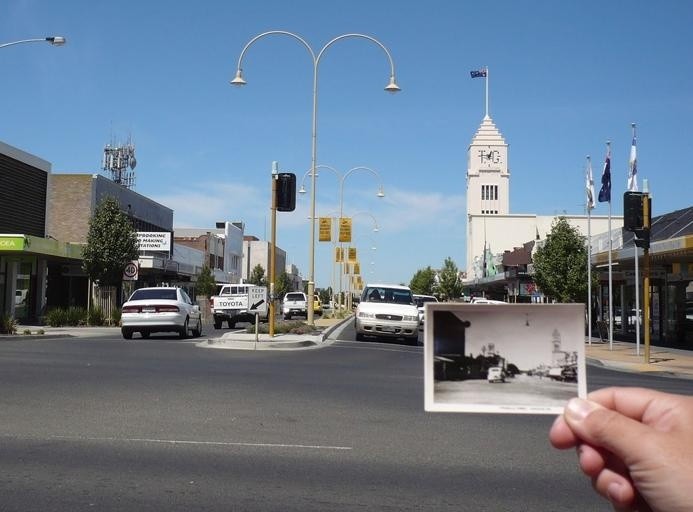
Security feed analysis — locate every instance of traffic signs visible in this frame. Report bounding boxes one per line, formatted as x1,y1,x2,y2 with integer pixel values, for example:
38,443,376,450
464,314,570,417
247,286,267,312
124,263,137,277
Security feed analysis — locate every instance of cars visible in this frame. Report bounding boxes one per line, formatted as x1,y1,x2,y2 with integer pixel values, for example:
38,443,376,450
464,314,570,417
14,287,28,305
487,367,507,384
352,284,510,345
120,287,202,340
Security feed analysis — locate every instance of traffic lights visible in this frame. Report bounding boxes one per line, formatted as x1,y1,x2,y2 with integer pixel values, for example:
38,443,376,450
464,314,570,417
635,222,650,249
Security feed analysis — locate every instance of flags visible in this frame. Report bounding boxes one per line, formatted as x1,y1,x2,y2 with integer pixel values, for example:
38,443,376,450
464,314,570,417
626,135,637,192
597,150,612,203
470,68,486,79
585,162,596,210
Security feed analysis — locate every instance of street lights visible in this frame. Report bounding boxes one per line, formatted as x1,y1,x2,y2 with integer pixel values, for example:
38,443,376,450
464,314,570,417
300,164,385,317
231,31,403,336
1,33,69,59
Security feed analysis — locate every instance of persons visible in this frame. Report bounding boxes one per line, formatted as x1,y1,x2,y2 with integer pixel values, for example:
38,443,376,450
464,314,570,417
548,384,693,512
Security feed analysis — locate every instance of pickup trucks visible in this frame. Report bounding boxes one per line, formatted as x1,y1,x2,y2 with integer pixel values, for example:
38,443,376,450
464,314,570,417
210,282,270,326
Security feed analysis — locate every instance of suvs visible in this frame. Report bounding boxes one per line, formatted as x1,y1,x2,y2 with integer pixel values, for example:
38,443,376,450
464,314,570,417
278,290,324,319
